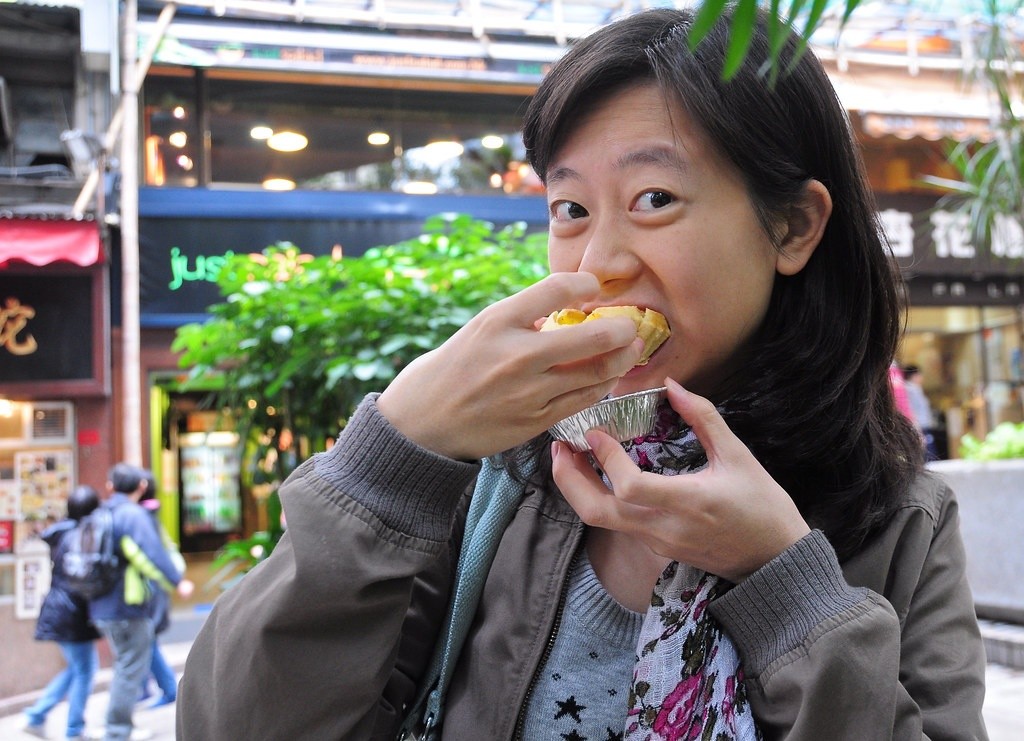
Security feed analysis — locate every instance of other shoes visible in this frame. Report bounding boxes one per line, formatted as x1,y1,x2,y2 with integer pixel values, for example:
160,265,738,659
65,735,99,741
149,694,176,709
21,723,53,741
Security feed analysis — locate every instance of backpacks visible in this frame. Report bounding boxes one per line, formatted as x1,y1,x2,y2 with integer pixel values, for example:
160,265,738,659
54,500,129,599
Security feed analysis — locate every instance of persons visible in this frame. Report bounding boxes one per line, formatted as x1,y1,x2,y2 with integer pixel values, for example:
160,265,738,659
903,366,933,460
24,464,195,741
175,9,985,741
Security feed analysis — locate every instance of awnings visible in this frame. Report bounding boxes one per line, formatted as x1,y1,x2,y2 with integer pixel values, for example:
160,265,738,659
0,218,106,269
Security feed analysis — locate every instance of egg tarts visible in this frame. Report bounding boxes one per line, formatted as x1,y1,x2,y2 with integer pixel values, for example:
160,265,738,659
535,306,672,366
